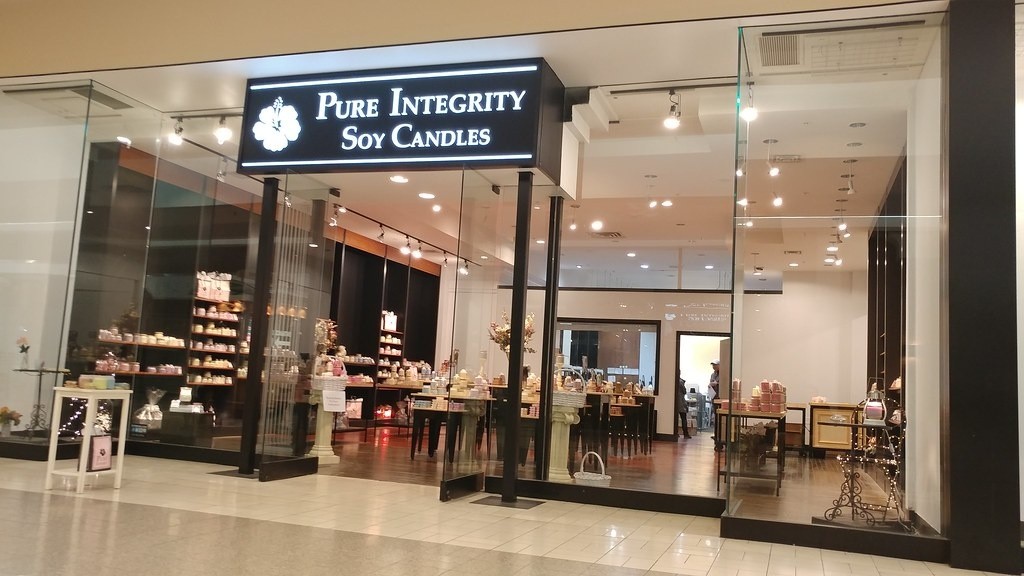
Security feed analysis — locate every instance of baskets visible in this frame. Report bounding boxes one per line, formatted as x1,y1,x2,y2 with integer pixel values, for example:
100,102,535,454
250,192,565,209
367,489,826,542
552,369,587,409
311,354,348,390
573,451,611,487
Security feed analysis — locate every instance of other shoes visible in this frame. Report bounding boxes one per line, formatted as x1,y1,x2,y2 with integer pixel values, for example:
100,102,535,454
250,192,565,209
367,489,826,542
683,435,691,439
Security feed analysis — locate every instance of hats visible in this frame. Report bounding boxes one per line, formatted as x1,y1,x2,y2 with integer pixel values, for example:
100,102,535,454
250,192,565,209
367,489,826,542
711,360,722,366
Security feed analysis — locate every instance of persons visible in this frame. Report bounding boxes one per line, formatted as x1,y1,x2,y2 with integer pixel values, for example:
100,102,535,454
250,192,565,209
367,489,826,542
708,358,721,443
676,369,692,439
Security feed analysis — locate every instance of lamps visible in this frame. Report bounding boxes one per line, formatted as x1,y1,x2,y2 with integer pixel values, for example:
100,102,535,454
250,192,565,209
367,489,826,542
168,117,185,146
377,224,385,242
834,255,842,266
442,251,448,268
738,82,759,123
400,236,412,255
459,260,470,276
338,207,347,214
835,232,843,245
848,159,856,196
844,230,851,239
836,201,848,231
412,240,423,258
663,89,683,131
211,116,232,147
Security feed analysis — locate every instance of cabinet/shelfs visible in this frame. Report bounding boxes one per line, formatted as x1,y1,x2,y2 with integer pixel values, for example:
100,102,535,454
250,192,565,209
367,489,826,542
65,148,441,438
